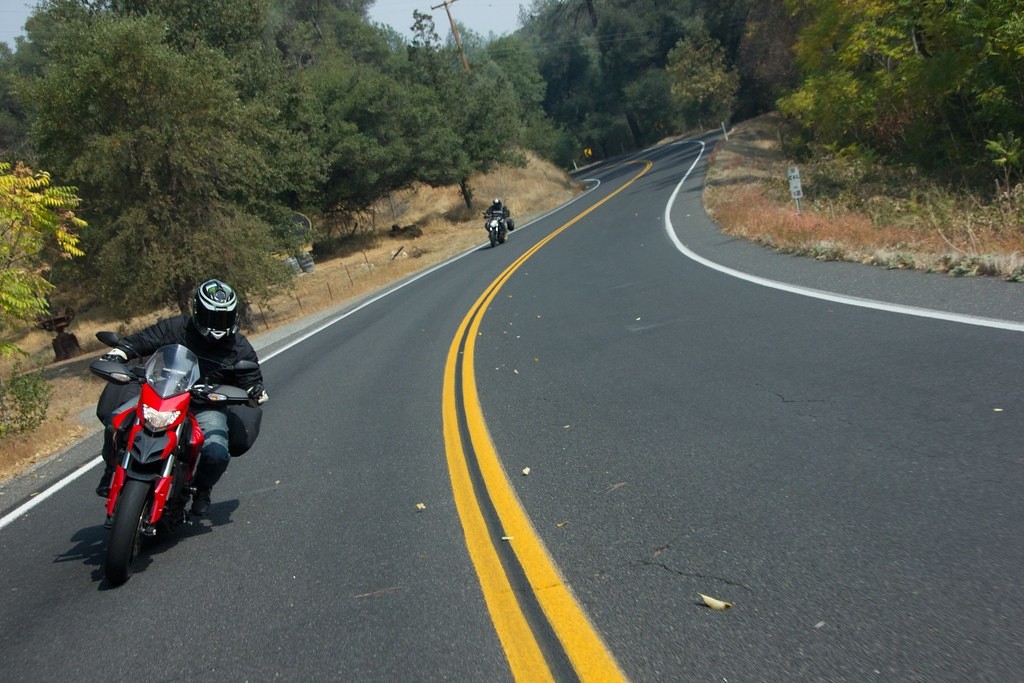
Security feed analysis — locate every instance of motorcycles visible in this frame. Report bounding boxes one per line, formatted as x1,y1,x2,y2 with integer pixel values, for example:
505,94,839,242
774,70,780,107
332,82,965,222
90,331,257,584
483,211,514,247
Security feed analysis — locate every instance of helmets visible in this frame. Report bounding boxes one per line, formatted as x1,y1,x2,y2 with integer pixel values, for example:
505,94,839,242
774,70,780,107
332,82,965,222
193,279,238,339
493,199,501,208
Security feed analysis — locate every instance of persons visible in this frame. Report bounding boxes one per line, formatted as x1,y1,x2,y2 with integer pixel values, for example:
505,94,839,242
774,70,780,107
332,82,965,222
484,198,508,240
100,279,269,515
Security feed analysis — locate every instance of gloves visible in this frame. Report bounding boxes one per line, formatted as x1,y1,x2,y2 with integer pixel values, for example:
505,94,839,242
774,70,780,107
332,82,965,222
99,347,129,364
245,384,269,403
503,215,507,218
484,216,487,219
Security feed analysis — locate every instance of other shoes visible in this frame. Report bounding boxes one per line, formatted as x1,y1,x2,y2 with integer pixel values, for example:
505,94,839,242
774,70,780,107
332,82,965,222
190,491,210,516
96,473,113,496
504,233,509,240
